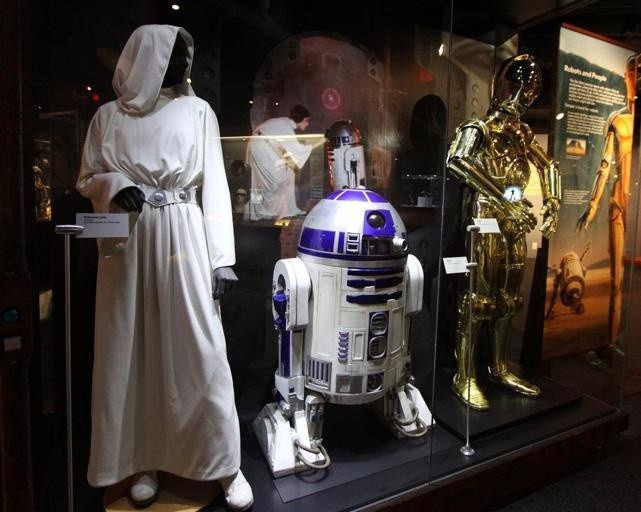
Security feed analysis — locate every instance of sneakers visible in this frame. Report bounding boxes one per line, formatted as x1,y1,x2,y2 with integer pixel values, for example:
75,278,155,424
128,471,162,507
220,469,256,509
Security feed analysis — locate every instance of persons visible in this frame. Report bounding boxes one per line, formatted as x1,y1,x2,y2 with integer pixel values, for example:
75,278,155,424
445,53,564,411
572,55,636,372
237,106,329,220
75,24,255,509
